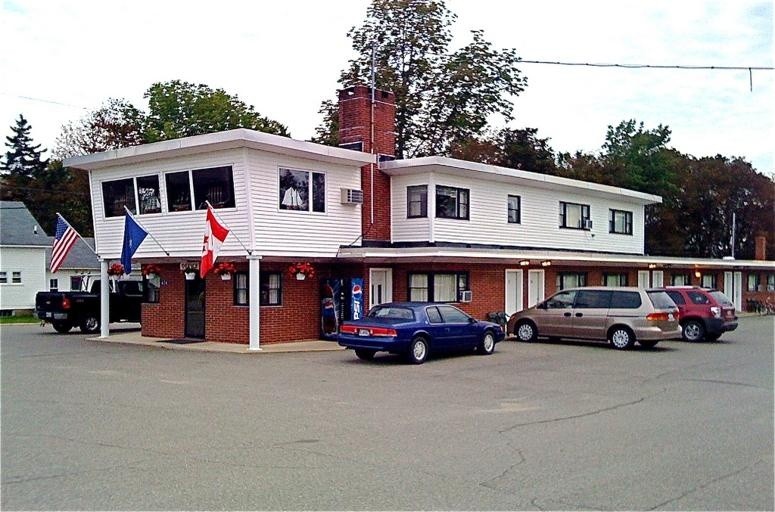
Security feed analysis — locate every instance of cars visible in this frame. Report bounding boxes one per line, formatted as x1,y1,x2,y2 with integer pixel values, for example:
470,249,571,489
665,285,739,343
338,300,505,364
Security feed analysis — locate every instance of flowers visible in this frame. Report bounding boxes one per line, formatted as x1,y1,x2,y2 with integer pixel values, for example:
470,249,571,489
213,262,237,277
107,263,124,276
141,263,160,275
286,260,316,278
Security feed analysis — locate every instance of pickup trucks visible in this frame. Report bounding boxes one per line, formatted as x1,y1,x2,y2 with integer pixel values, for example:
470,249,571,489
35,279,161,336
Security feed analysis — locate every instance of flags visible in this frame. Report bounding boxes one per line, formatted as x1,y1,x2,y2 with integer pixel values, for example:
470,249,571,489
121,212,149,275
49,217,79,274
199,208,231,278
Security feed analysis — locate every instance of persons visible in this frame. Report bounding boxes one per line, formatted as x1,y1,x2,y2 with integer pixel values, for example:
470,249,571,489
764,295,773,312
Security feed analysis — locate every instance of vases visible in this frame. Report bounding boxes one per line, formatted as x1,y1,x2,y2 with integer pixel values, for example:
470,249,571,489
221,272,231,280
111,274,121,280
146,273,155,279
295,272,306,280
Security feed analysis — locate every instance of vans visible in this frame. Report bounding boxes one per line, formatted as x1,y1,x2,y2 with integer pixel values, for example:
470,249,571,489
506,286,683,349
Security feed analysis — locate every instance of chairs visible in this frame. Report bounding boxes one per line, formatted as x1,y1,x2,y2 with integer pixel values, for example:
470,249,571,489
487,313,505,332
497,311,510,332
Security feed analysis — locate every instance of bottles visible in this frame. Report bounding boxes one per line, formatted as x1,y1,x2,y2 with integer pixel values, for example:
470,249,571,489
321,281,336,335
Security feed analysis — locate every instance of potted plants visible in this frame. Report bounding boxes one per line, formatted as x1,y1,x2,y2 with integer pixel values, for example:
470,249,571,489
182,268,199,280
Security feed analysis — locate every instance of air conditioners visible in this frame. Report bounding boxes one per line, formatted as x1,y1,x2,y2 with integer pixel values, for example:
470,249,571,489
583,220,593,229
459,291,472,303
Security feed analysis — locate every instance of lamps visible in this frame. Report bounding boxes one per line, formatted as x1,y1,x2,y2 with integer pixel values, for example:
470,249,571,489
695,272,701,281
519,260,530,266
540,260,552,268
648,263,657,269
